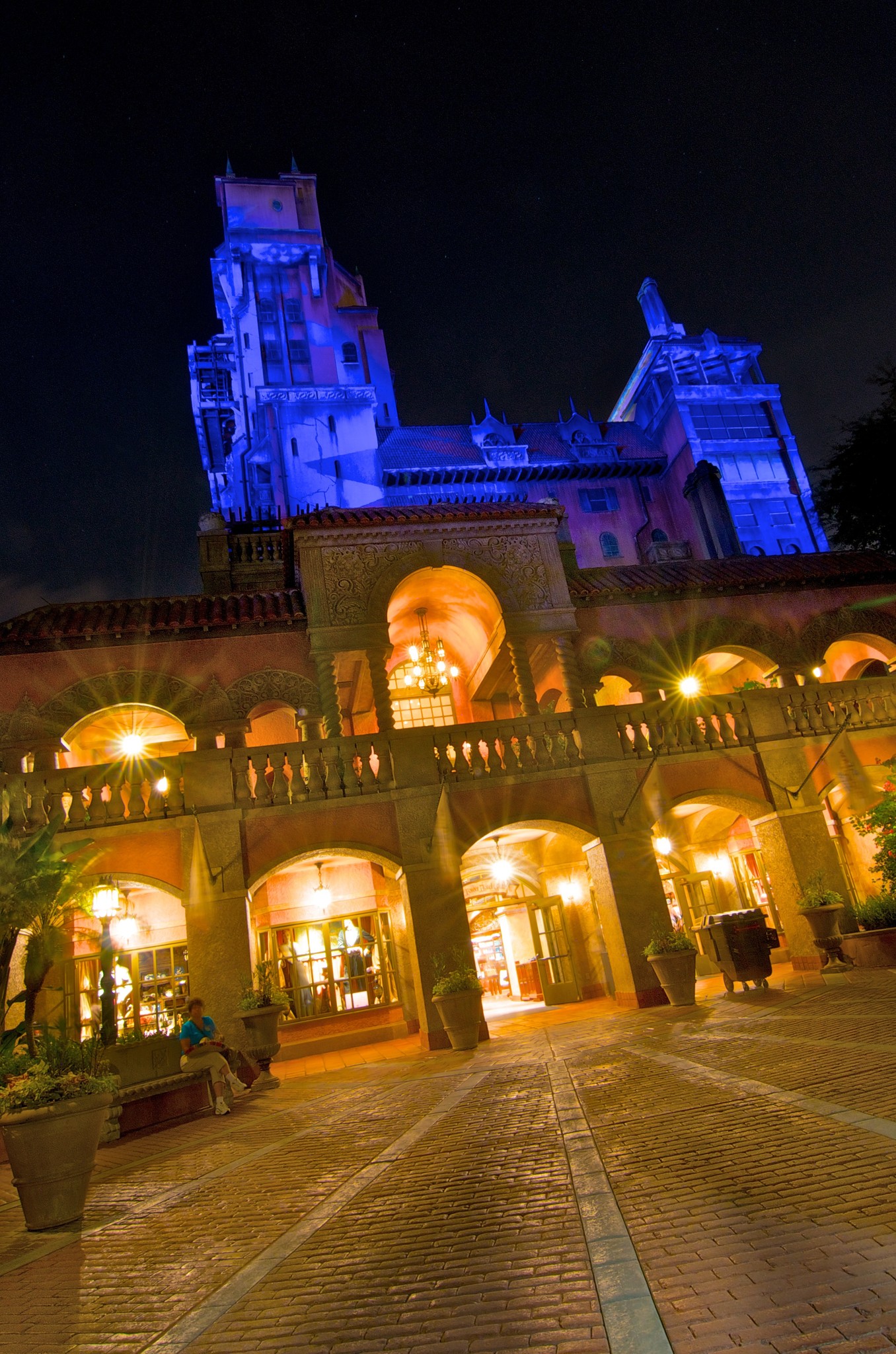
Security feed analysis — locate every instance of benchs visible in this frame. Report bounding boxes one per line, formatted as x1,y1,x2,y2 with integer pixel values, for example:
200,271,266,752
99,1060,241,1142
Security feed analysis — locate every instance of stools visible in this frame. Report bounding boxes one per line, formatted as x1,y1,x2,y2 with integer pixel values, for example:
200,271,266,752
488,975,502,996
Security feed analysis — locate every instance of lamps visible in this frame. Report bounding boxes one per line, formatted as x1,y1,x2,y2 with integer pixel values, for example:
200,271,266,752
404,607,459,699
314,862,326,891
117,889,137,946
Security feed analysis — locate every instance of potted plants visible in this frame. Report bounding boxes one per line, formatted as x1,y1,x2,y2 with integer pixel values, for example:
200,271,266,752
0,1059,120,1230
641,913,698,1006
429,946,488,1050
791,870,853,974
233,959,293,1091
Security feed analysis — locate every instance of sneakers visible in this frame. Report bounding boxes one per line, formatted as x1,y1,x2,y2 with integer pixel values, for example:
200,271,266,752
215,1102,230,1115
231,1078,248,1091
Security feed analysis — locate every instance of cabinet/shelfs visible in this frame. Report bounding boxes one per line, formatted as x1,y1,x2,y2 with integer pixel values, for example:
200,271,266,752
515,960,552,1002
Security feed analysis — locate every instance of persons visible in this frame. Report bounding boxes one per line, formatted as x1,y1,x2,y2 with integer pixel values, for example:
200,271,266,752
180,996,248,1115
98,956,132,1037
280,929,315,1018
337,918,375,994
